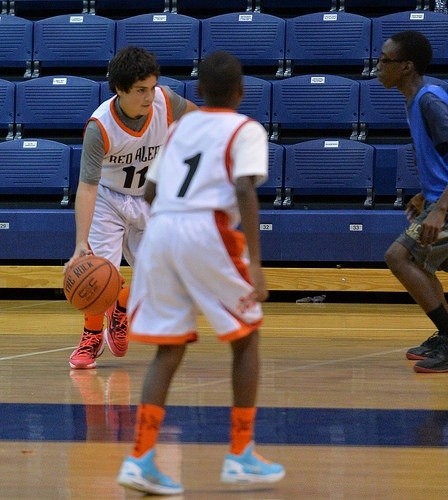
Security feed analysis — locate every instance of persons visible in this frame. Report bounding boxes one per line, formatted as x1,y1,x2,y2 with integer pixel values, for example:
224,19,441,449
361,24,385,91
60,45,199,371
114,47,290,495
373,30,448,373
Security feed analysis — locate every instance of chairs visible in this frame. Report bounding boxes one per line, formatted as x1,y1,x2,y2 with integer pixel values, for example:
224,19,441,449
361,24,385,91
0,0,448,209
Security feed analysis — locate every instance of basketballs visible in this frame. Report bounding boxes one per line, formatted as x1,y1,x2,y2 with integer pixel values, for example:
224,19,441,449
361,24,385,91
63,256,120,314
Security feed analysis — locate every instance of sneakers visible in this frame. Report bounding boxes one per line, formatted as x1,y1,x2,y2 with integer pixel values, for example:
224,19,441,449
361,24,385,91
69,324,105,369
103,287,131,357
70,369,110,406
414,342,448,373
119,449,184,494
220,442,286,484
105,371,134,426
406,330,441,359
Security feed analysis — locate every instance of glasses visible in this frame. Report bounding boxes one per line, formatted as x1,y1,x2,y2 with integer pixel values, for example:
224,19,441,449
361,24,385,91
377,52,404,68
